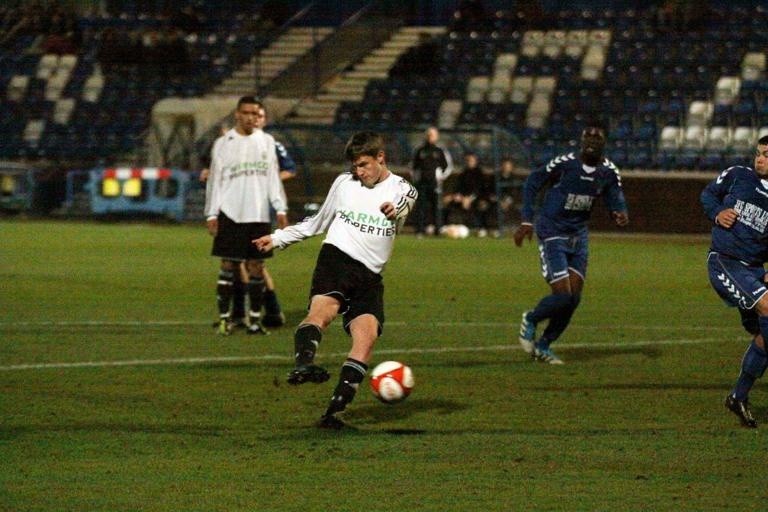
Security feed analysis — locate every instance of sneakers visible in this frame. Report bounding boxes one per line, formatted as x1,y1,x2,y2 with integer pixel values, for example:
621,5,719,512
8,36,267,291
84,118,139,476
518,311,535,356
225,313,250,328
725,392,759,428
285,363,331,385
260,312,285,327
248,310,261,335
211,313,233,336
534,347,564,366
316,414,346,430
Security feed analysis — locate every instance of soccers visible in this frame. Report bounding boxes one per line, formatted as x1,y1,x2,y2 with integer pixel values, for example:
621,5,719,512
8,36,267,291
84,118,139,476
370,361,415,405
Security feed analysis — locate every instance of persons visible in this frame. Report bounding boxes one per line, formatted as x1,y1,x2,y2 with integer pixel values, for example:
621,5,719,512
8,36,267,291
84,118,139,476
440,151,523,239
516,125,630,366
410,129,453,234
701,136,768,429
251,132,418,411
199,102,296,326
255,104,298,182
204,97,289,336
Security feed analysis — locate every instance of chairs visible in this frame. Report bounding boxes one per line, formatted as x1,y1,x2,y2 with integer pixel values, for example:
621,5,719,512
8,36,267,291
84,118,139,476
497,54,516,68
589,31,600,43
759,128,768,139
565,46,580,56
55,99,75,109
87,76,102,88
588,45,602,55
743,53,765,80
698,155,723,172
665,154,679,171
467,92,481,102
510,91,526,103
53,113,68,124
441,101,461,115
567,31,578,44
84,89,98,102
600,31,611,44
57,70,68,79
732,127,757,151
489,91,504,102
723,155,747,170
512,78,532,90
544,33,554,43
469,78,488,90
532,31,543,43
581,69,598,80
577,31,586,44
524,31,532,43
7,90,20,101
533,93,548,99
535,77,555,89
526,116,543,128
716,77,741,105
37,69,50,78
661,126,683,148
39,134,63,158
684,126,707,149
494,69,510,79
583,55,603,66
492,79,510,90
11,77,27,87
530,100,549,111
439,116,454,128
709,127,731,150
46,90,60,101
522,46,538,56
554,31,565,43
59,56,76,69
679,154,698,172
48,78,64,88
543,46,558,56
23,121,43,140
41,56,58,67
688,101,714,126
